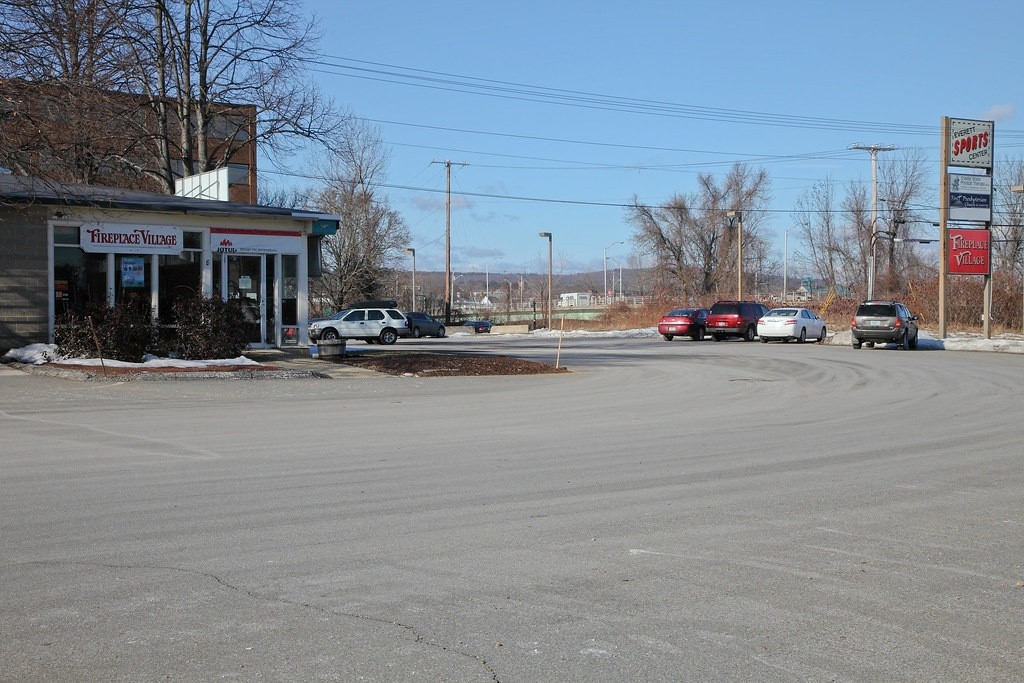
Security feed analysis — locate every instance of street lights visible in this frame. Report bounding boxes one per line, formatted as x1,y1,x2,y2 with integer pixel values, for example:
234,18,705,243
727,211,743,302
404,247,416,312
783,221,808,303
537,231,551,332
606,257,622,301
604,240,625,306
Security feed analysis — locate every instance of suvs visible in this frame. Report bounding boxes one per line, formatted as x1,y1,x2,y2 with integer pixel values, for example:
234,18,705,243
850,299,920,351
307,300,410,346
706,301,770,342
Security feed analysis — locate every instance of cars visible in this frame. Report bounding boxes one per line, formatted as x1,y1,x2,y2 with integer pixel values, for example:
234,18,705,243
474,322,491,333
404,312,446,338
757,308,826,343
657,308,712,341
